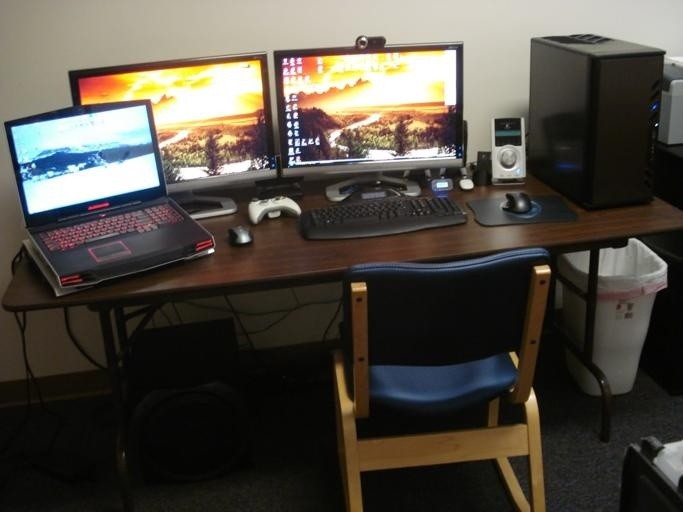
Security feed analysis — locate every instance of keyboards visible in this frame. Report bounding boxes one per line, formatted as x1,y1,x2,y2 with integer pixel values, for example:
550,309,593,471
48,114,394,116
299,195,467,241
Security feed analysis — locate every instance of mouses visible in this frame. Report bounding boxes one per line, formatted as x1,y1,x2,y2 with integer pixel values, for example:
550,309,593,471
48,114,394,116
228,224,254,246
506,190,533,214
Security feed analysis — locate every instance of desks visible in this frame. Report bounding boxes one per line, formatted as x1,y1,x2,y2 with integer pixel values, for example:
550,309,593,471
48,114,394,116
1,170,683,512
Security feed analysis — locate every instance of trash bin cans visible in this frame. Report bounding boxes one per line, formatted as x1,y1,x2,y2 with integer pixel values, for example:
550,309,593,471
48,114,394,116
559,238,668,397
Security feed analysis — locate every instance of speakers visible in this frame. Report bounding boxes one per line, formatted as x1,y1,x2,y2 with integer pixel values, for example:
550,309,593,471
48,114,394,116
529,34,666,212
491,116,527,186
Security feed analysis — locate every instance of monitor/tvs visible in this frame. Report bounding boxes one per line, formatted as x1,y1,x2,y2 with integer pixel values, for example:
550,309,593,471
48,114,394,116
67,51,278,221
274,42,465,202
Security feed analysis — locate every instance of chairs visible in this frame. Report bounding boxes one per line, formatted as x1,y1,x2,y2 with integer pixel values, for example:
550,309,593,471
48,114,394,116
330,246,558,512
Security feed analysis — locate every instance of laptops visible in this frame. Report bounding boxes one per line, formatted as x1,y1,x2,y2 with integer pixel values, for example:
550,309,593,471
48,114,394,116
4,99,217,289
